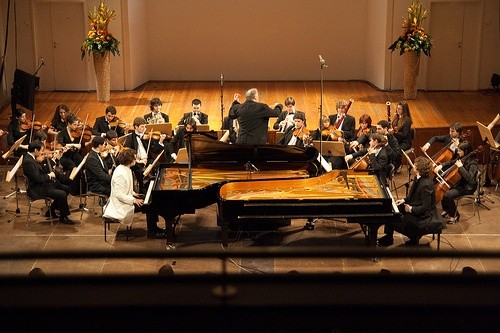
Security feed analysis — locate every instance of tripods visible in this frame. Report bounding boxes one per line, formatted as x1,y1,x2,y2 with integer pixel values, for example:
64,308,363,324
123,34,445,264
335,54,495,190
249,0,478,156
460,121,500,210
3,133,97,222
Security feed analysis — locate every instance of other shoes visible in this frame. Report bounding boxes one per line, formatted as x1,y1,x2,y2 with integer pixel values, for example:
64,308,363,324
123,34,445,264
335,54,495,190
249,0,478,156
379,235,393,245
405,238,419,247
147,227,167,239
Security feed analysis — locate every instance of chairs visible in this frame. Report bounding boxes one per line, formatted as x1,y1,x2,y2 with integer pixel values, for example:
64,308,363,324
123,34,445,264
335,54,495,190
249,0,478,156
22,173,54,228
79,169,105,222
400,128,417,172
455,169,481,221
98,195,131,242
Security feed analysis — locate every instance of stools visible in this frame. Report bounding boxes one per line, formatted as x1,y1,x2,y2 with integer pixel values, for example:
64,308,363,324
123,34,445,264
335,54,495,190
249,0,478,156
422,209,445,251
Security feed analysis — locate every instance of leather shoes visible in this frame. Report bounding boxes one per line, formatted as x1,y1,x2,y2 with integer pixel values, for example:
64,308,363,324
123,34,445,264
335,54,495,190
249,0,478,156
59,217,75,225
44,212,60,218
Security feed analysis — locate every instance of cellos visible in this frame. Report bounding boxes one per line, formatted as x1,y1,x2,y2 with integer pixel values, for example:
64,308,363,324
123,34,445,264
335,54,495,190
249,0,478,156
488,146,500,192
434,145,484,205
432,129,472,179
349,139,388,170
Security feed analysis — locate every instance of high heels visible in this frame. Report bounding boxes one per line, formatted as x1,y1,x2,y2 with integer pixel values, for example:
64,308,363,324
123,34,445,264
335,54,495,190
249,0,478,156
441,212,448,217
447,214,460,224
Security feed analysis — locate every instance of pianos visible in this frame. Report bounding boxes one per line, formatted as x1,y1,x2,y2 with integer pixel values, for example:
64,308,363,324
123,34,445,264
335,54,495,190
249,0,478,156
141,144,319,245
217,169,403,248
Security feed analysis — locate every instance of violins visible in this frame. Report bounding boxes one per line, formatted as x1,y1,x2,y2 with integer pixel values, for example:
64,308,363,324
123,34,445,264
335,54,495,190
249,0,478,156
70,127,92,140
22,119,55,129
142,130,174,140
36,142,130,163
108,117,133,128
322,125,349,145
356,128,371,138
293,127,313,143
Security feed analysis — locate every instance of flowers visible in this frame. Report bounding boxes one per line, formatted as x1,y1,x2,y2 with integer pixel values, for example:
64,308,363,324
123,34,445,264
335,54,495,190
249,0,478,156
78,0,123,65
387,0,435,61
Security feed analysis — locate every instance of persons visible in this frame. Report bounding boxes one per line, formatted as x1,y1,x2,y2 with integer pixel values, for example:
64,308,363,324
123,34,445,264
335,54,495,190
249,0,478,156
220,87,283,145
7,104,93,196
309,99,401,183
388,101,411,174
85,105,197,196
102,148,167,238
175,98,208,129
23,141,74,224
422,122,479,223
278,113,312,147
377,157,436,245
273,96,306,132
144,97,169,124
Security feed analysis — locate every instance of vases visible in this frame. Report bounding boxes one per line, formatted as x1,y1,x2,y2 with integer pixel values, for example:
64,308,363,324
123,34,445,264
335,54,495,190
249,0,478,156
403,50,418,99
91,50,112,104
